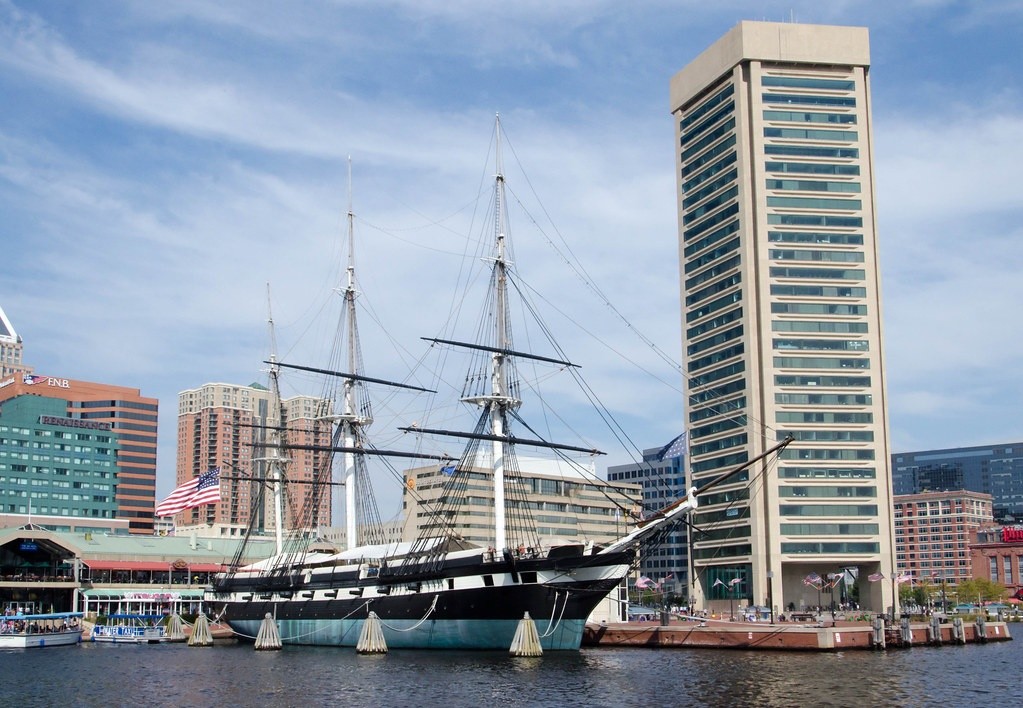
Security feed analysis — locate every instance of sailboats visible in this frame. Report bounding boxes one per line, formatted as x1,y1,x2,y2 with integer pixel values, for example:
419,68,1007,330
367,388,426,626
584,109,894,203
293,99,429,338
199,112,798,660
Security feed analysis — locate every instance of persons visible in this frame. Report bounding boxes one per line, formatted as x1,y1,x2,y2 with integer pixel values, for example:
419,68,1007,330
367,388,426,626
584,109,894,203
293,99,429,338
0,616,79,635
644,600,943,630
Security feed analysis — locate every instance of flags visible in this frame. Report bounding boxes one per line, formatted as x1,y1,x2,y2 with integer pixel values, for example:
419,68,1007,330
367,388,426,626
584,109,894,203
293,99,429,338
632,567,944,594
156,466,222,517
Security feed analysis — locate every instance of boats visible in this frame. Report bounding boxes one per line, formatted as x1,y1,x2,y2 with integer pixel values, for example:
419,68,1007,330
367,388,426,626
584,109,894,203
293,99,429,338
0,611,84,649
89,614,172,645
181,623,236,639
